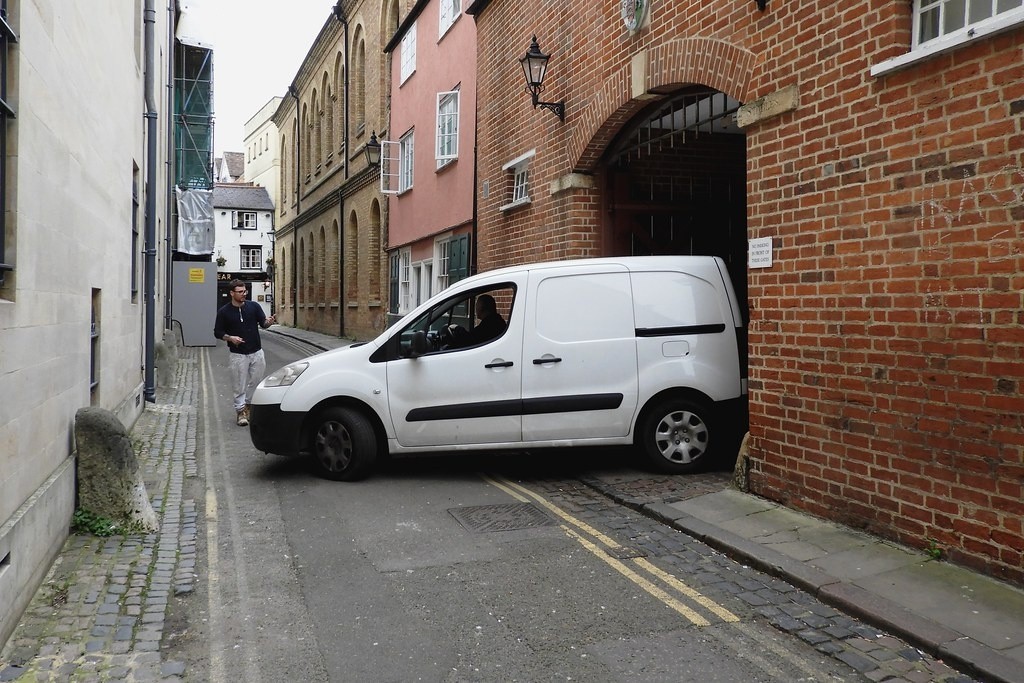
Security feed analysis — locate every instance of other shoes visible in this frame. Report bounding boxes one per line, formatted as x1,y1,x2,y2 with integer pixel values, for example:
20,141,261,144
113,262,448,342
245,404,250,420
237,411,249,426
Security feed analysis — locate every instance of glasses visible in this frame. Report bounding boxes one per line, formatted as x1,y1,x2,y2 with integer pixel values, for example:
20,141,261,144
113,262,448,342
234,290,249,295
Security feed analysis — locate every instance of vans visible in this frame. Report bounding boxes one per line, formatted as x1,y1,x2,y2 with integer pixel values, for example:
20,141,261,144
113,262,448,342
248,256,746,481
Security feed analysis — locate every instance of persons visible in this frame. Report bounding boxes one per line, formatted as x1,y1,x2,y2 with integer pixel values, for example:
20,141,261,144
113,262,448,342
214,279,276,426
448,294,507,351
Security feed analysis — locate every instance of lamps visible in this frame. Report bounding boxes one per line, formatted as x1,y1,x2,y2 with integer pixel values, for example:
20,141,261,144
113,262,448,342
363,132,382,166
518,35,566,122
